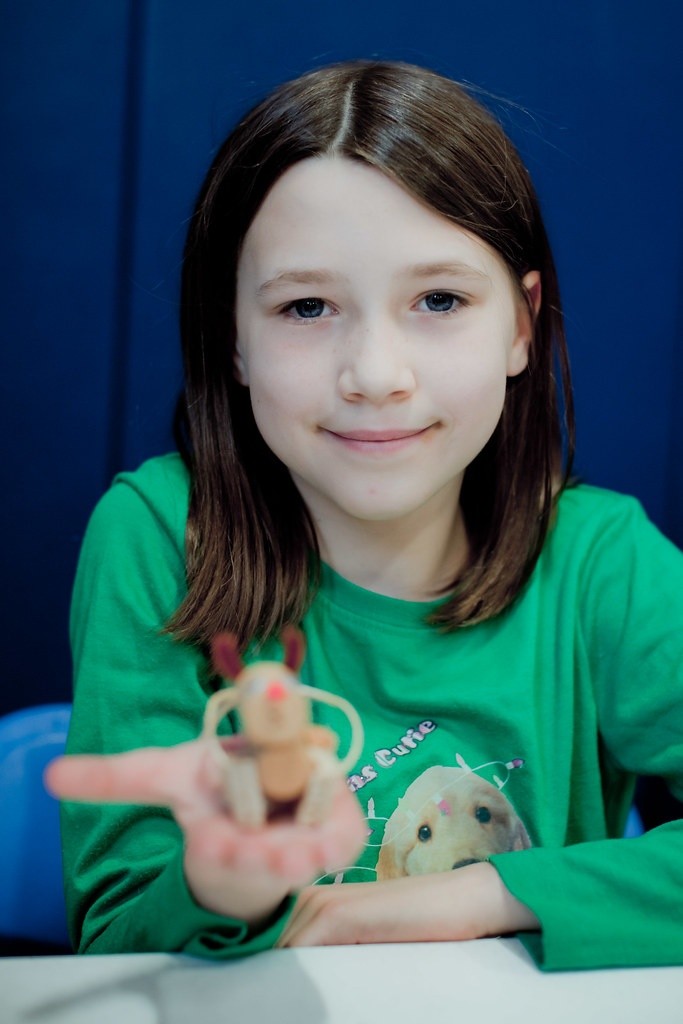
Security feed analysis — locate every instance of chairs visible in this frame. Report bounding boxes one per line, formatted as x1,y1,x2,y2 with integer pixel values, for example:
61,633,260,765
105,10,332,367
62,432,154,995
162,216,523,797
0,704,78,957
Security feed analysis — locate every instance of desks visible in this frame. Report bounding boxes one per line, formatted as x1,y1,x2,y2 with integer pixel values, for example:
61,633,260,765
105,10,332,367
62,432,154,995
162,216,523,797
0,938,683,1024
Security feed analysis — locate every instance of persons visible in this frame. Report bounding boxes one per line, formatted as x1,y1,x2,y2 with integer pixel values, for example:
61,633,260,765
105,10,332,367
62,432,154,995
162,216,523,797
46,60,683,971
